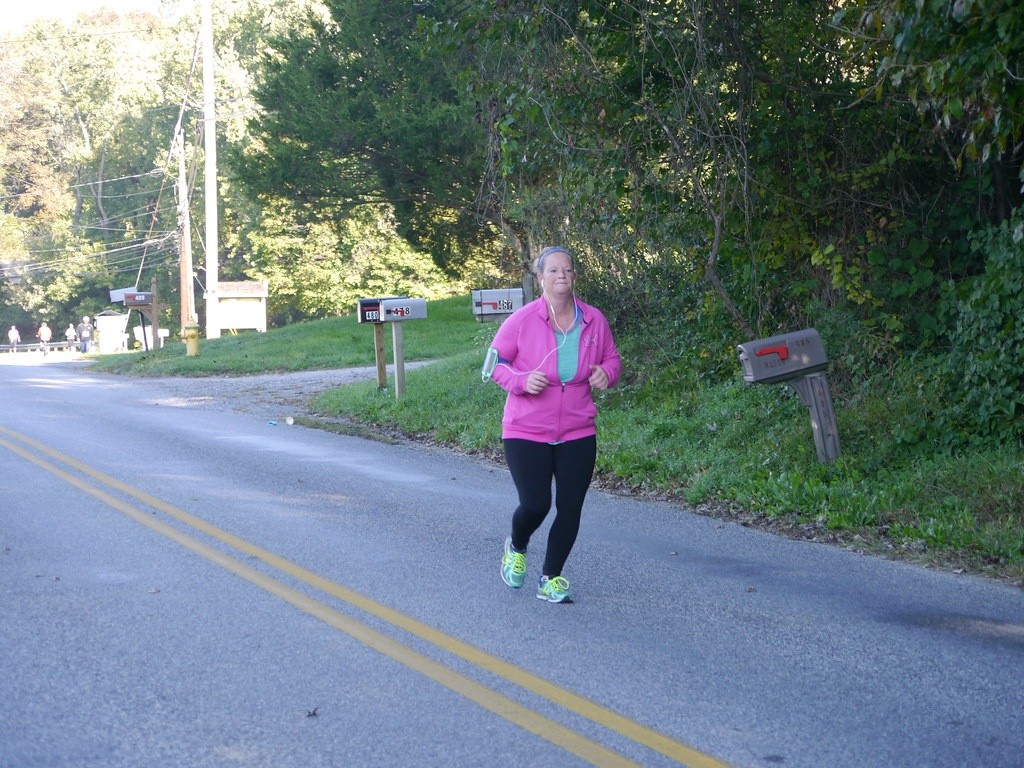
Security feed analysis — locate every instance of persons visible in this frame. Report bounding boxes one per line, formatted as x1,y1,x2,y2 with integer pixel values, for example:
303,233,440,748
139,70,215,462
65,323,76,351
38,322,52,354
481,247,620,603
8,325,21,354
76,316,94,354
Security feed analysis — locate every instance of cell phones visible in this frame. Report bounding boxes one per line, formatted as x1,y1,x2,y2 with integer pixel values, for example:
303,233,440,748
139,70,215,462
482,347,497,378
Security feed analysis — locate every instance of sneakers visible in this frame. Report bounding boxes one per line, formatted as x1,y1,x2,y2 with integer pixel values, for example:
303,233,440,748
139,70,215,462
537,575,572,603
500,537,527,589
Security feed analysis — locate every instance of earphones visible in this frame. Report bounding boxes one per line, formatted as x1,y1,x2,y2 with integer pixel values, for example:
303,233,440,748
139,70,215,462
541,279,545,288
573,280,575,285
